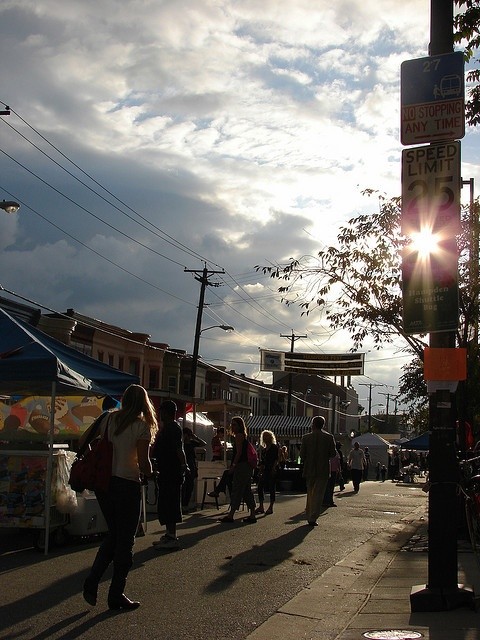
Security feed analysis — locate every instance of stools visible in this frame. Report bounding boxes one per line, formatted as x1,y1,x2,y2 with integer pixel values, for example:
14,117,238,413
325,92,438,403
200,476,221,510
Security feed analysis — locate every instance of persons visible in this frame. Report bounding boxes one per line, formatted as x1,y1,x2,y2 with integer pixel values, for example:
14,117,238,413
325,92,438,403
210,424,226,462
299,415,336,526
150,399,190,552
335,441,345,491
77,386,156,613
218,416,258,523
379,465,388,481
389,446,429,483
254,430,280,515
323,445,340,509
281,445,289,465
207,436,237,500
181,427,207,512
347,442,367,493
363,447,372,481
101,395,119,416
375,462,381,480
1,414,23,451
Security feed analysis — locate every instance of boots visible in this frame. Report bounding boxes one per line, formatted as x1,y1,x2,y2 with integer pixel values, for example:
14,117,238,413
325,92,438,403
107,575,141,611
83,552,108,606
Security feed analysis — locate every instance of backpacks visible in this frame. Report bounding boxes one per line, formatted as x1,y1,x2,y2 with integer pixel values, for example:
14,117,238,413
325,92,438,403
159,446,190,483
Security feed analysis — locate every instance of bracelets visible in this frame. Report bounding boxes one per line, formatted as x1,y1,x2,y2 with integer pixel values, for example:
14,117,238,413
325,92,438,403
181,463,189,467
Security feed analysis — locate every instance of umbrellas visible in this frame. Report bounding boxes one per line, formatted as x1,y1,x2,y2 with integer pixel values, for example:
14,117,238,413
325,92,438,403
400,433,431,450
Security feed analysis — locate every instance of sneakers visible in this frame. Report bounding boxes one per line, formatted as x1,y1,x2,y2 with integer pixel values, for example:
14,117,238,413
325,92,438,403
255,507,264,514
266,507,273,514
243,516,256,523
207,491,219,497
328,503,337,507
308,522,318,526
218,516,234,522
152,534,182,551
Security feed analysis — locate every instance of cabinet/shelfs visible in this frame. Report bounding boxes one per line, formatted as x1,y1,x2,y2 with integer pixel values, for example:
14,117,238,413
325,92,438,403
194,397,254,504
144,387,201,515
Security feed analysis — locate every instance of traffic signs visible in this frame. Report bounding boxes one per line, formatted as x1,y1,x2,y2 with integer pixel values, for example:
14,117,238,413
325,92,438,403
401,139,462,234
401,51,465,146
401,236,460,334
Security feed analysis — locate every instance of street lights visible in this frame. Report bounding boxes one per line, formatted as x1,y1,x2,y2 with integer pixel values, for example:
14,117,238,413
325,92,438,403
190,325,234,397
0,199,20,215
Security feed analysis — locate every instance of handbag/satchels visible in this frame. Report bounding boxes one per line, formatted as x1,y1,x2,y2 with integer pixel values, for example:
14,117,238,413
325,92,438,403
68,439,113,492
246,441,257,469
